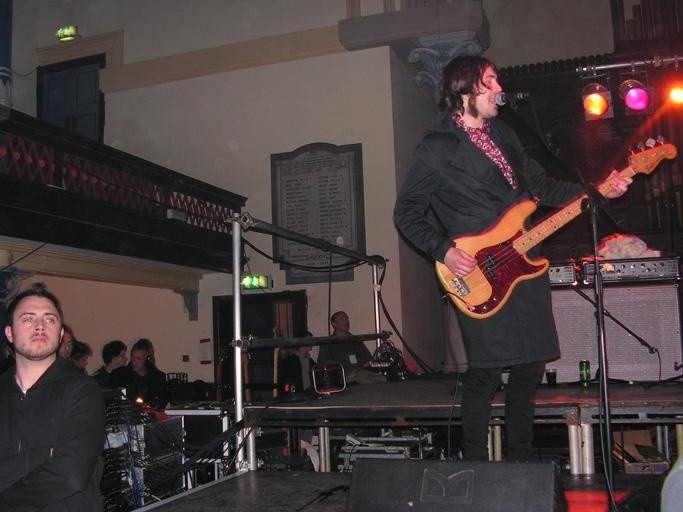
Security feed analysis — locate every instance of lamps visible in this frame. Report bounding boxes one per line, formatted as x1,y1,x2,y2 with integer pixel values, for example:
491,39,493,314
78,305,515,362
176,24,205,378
579,54,683,125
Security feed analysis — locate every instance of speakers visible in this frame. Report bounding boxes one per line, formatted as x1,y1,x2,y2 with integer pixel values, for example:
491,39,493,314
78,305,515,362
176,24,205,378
345,457,569,512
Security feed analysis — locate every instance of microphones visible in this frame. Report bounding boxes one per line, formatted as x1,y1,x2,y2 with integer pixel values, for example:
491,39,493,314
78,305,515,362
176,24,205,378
495,92,530,107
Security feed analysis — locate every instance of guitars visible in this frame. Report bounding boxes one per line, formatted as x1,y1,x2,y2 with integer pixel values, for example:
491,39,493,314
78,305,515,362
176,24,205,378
435,135,677,319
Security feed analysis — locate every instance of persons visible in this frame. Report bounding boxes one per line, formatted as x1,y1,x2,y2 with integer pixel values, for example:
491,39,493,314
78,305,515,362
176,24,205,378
0,293,103,512
55,326,74,360
318,311,372,371
279,332,316,444
71,342,91,370
393,56,634,461
92,340,130,386
111,338,170,412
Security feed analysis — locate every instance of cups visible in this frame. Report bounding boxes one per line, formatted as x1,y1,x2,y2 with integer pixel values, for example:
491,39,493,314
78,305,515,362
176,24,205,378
545,368,557,389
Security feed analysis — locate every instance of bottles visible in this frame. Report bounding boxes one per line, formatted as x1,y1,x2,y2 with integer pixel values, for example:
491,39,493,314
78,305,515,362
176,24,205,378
578,359,591,387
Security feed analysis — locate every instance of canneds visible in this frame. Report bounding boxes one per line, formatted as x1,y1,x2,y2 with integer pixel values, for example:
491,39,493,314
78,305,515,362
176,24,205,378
579,359,590,384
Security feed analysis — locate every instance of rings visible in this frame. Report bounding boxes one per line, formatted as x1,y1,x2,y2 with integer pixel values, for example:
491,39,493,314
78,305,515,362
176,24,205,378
456,273,460,278
619,189,622,195
